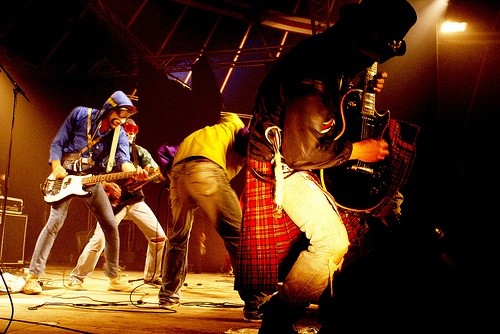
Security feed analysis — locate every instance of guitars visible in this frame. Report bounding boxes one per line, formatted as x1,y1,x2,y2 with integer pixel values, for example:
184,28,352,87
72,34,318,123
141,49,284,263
106,168,162,208
39,165,155,204
319,62,394,212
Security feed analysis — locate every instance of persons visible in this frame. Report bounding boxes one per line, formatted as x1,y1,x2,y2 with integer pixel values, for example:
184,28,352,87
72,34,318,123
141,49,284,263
69,120,167,290
159,111,266,323
23,91,150,293
239,0,419,334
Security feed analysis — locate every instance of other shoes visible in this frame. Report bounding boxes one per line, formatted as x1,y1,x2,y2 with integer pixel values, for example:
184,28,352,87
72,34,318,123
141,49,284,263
108,277,139,292
159,299,179,307
68,283,83,290
243,310,263,321
144,277,163,287
22,278,42,294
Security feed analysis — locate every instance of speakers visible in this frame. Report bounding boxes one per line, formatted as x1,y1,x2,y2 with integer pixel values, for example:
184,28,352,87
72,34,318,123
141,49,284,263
0,213,28,264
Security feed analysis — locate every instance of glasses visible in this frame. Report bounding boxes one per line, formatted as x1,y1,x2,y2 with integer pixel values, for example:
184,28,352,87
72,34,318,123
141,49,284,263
120,124,140,135
116,108,132,118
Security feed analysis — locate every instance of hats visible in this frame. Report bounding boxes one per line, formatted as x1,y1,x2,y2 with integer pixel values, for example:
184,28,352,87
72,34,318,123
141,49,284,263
338,0,417,64
